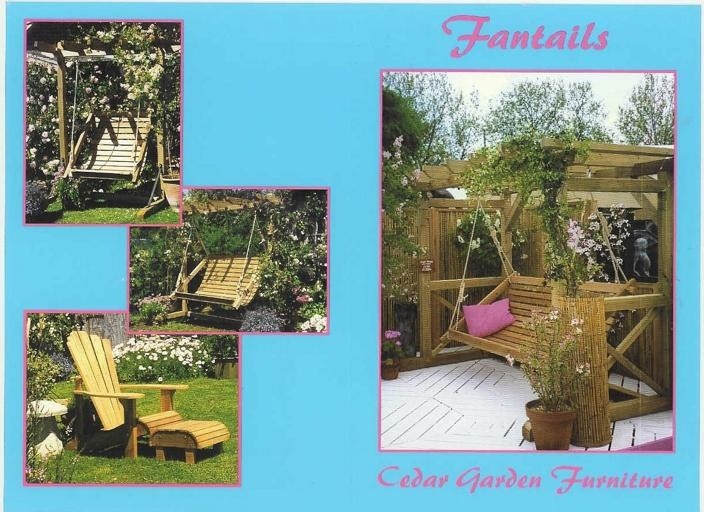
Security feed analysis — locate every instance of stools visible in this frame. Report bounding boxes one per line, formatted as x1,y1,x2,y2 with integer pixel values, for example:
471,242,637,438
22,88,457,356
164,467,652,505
147,419,231,464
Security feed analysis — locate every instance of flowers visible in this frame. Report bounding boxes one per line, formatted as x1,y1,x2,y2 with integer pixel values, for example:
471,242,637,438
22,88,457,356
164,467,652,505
453,208,529,274
566,200,631,282
381,329,405,360
504,308,594,409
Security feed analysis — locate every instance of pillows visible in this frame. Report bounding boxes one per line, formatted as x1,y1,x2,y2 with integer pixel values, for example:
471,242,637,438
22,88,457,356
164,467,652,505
461,297,516,337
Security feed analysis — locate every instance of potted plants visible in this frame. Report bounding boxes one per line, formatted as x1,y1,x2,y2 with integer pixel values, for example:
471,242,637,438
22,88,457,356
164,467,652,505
153,49,179,214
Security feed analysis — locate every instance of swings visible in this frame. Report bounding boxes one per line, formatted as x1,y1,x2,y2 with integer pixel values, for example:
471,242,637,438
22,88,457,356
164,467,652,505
432,198,635,366
62,61,153,184
171,208,268,310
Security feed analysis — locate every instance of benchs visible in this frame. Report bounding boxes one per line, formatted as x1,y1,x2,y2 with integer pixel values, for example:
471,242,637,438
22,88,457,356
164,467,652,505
433,270,636,372
59,107,150,184
165,256,261,328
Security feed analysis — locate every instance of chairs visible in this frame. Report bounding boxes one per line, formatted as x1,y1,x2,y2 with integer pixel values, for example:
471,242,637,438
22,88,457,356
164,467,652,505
64,328,188,464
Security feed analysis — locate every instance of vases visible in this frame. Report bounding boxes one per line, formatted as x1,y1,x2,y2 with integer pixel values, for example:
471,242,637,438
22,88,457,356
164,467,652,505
381,360,405,380
524,398,578,450
